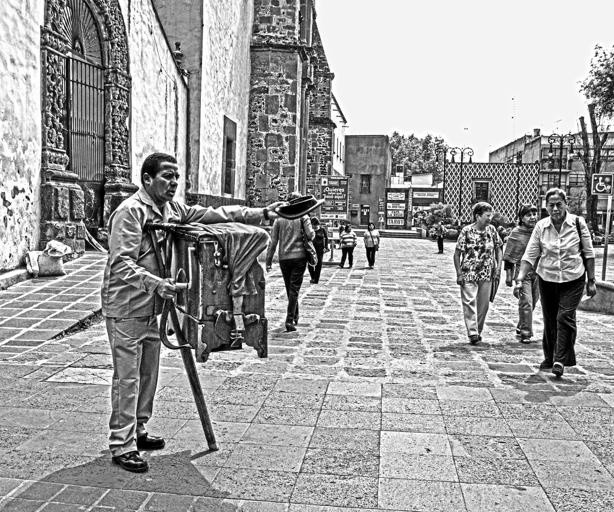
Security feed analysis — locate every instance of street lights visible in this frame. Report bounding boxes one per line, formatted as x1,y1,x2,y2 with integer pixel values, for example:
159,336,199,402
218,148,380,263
435,146,452,204
548,133,575,189
449,147,474,217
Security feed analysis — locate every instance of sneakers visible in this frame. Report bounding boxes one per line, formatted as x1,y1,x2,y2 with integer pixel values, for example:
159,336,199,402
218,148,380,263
515,327,522,336
522,337,530,344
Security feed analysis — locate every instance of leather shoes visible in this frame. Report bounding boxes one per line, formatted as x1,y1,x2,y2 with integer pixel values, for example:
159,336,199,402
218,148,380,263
114,452,148,472
552,362,564,377
131,435,164,447
541,360,553,369
469,336,477,343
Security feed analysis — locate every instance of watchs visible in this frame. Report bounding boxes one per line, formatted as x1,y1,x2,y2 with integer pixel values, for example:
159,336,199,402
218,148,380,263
588,278,596,283
263,209,270,220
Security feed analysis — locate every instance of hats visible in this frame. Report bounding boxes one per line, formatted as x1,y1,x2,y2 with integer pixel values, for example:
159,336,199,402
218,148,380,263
271,196,326,219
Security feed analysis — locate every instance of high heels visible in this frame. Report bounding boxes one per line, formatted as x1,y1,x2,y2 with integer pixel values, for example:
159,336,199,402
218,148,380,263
295,318,299,327
285,323,296,331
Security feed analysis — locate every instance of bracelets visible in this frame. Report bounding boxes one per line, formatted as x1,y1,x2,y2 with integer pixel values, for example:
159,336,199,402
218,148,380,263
457,274,463,277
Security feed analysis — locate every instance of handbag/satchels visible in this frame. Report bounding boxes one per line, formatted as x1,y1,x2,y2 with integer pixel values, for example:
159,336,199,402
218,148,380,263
302,219,317,270
368,233,380,249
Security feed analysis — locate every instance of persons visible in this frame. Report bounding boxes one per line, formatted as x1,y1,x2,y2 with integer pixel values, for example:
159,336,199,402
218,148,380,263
338,220,345,249
266,192,318,331
437,221,449,254
307,218,328,283
513,187,596,377
504,204,541,343
364,223,380,268
101,153,292,473
454,201,504,344
339,224,357,269
